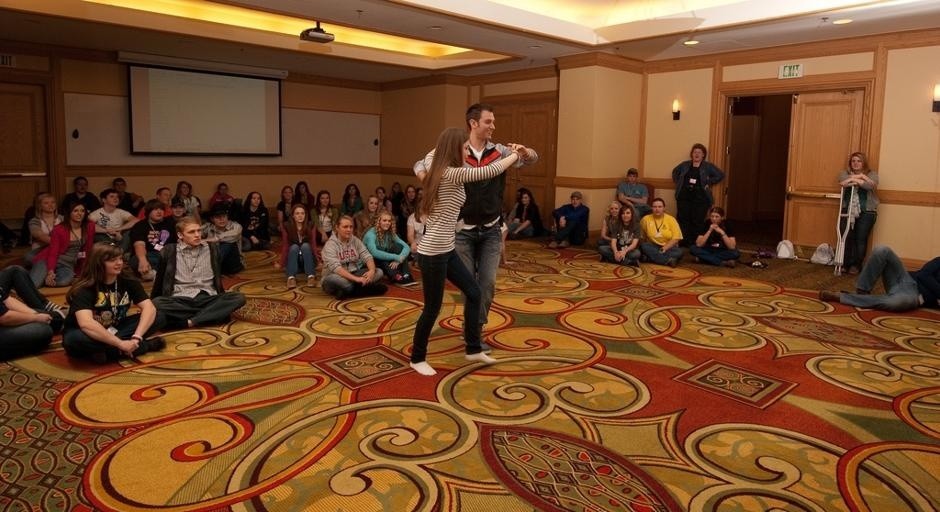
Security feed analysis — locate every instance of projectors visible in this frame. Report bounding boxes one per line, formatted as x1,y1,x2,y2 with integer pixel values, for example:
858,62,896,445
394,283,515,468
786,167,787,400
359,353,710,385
300,27,334,44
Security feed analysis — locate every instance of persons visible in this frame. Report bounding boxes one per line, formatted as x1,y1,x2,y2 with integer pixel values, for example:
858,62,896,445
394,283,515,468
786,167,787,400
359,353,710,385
500,144,740,269
20,177,275,288
819,245,940,313
410,127,521,376
413,103,538,356
61,242,167,363
0,265,65,360
277,180,429,298
838,152,879,275
149,217,247,333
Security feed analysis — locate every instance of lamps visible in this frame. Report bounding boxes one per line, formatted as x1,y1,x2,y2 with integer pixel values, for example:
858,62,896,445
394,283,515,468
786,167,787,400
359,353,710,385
672,99,680,120
932,83,940,112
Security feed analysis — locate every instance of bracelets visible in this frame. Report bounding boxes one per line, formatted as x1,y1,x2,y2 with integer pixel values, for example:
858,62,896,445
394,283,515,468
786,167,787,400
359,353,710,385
131,335,143,343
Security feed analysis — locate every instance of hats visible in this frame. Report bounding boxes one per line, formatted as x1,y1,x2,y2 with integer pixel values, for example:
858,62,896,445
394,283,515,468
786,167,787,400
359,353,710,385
571,191,582,199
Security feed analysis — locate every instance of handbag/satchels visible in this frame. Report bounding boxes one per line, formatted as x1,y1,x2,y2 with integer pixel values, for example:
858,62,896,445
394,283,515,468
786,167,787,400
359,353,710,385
776,240,798,260
810,243,835,266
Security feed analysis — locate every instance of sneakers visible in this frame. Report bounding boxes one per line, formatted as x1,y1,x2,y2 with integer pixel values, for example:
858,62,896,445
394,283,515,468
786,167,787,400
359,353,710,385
695,257,703,264
548,239,570,249
307,274,317,287
627,258,640,267
287,276,297,288
841,266,859,276
391,273,420,287
819,289,849,303
664,256,678,268
720,259,736,268
464,339,492,354
145,336,166,352
599,253,609,262
